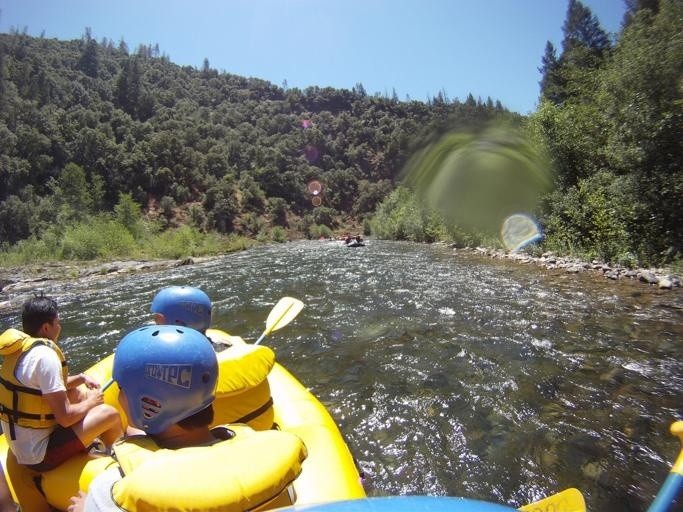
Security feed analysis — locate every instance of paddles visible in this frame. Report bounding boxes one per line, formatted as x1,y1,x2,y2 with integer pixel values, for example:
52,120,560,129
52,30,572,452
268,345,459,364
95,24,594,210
252,296,304,344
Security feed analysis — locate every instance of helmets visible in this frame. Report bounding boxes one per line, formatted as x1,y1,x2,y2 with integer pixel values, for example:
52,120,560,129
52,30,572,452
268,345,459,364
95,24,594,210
109,325,220,432
147,283,212,333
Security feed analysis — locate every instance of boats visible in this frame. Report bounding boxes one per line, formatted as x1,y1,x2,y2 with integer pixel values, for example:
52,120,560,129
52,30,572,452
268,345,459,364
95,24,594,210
348,240,365,248
0,327,366,512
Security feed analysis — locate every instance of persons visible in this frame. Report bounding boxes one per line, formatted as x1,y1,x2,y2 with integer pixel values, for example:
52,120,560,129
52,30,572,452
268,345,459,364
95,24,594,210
0,297,126,474
62,323,306,511
149,284,279,433
